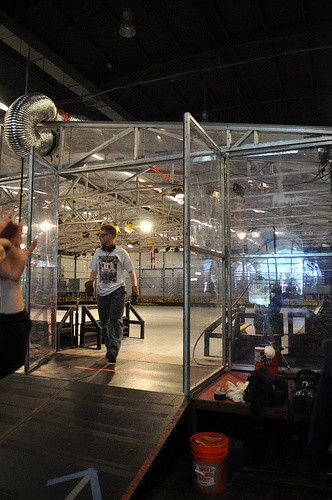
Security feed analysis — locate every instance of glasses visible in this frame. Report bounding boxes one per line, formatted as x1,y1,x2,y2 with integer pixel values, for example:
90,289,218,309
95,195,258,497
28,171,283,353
96,232,110,238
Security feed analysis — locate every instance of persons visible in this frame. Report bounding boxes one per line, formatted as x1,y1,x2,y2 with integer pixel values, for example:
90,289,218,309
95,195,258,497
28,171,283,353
84,224,140,363
0,217,38,378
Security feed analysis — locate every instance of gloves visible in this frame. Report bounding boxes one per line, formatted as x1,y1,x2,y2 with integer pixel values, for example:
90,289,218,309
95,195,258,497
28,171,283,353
85,281,95,297
131,286,140,304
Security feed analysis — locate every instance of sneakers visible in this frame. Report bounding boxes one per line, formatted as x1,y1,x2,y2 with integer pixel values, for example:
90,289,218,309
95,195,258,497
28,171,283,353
105,350,116,363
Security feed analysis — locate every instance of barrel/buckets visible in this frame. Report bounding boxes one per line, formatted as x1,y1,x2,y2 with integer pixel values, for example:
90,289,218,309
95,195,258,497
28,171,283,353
255,347,278,378
191,433,228,492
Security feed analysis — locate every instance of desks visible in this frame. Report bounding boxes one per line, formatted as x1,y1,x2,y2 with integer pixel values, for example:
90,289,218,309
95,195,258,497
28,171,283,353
191,371,300,435
